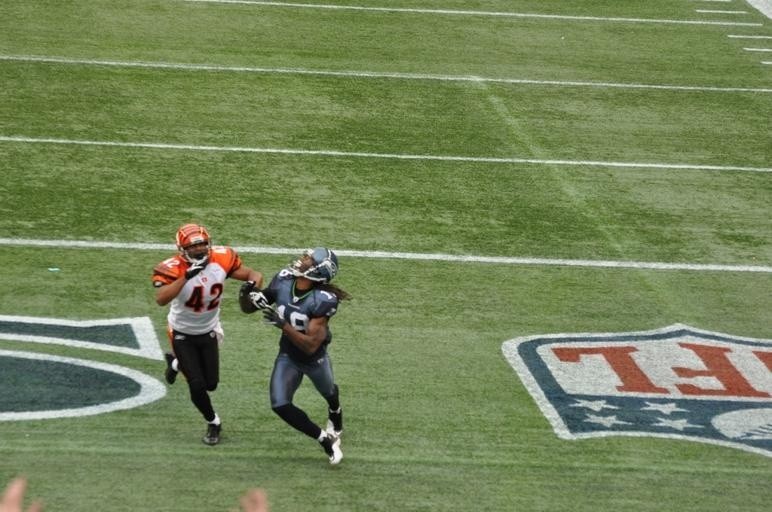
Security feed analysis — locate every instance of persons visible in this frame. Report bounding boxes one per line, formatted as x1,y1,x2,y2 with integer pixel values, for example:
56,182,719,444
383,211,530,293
1,475,272,512
152,224,264,445
238,246,344,466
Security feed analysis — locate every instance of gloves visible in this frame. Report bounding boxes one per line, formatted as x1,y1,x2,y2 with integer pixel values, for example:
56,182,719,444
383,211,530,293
185,264,204,279
239,280,286,329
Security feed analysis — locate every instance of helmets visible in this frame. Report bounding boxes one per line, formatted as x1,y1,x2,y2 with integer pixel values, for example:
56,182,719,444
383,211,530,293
287,246,339,282
176,224,212,267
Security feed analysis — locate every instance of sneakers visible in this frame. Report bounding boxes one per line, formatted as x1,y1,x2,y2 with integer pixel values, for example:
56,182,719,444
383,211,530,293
202,423,222,445
320,408,344,465
165,353,178,384
